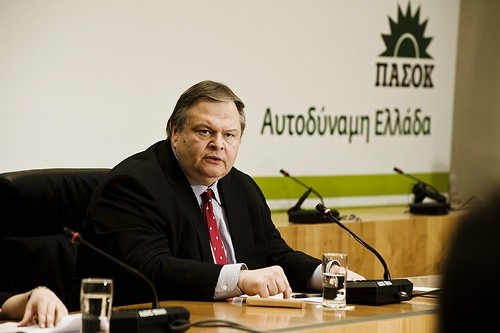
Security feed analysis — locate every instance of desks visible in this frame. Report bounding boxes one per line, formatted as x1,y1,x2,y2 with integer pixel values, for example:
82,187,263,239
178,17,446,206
67,203,468,333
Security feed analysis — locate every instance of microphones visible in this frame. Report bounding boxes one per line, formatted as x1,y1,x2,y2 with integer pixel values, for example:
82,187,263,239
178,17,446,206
394,167,450,214
64,227,189,333
279,170,341,223
315,204,414,306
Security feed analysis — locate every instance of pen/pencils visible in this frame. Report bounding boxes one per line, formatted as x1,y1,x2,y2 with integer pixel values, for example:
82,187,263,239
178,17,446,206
291,294,322,299
223,297,245,301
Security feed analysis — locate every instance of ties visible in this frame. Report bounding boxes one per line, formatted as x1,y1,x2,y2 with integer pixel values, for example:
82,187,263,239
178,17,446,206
199,189,227,265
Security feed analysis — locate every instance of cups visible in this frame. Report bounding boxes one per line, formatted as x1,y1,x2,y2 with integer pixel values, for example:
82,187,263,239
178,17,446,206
321,253,348,308
80,278,113,333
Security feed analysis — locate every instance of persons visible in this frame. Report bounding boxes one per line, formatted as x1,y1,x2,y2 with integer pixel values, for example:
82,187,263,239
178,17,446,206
73,80,366,312
0,285,68,328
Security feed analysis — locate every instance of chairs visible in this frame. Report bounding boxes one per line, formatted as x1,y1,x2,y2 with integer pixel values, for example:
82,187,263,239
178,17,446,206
0,168,111,314
